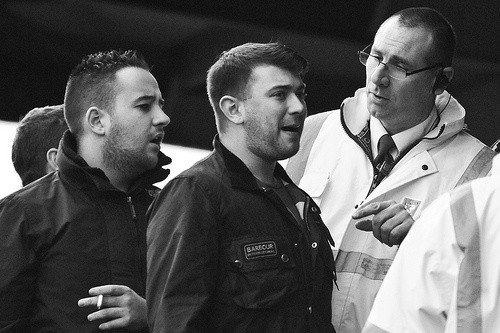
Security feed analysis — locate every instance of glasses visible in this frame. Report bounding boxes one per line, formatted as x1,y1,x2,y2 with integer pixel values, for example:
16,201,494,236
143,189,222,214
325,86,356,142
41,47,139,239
357,42,441,79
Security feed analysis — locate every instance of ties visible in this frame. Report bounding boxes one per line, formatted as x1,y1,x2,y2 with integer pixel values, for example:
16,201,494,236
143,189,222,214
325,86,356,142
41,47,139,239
369,135,397,189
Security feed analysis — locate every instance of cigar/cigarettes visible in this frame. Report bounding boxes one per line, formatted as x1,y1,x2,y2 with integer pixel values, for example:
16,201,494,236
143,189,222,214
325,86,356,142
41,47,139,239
97,295,103,309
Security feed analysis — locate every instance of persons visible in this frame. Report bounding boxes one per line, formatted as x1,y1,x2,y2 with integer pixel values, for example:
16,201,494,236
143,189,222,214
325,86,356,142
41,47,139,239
279,6,500,333
11,103,69,187
0,51,172,333
144,43,340,333
363,174,500,333
